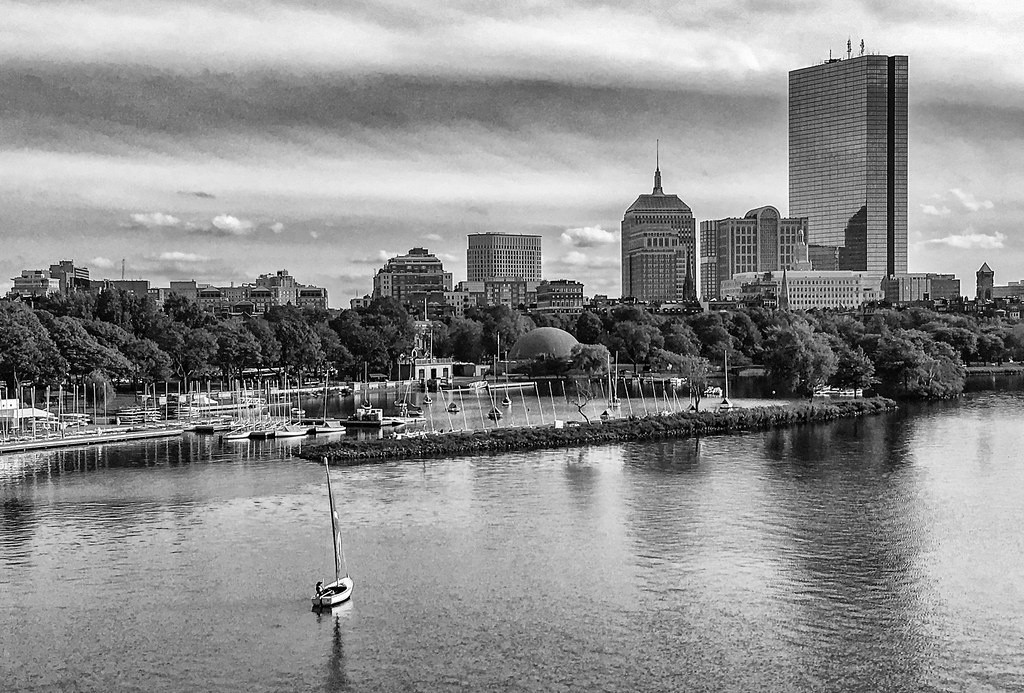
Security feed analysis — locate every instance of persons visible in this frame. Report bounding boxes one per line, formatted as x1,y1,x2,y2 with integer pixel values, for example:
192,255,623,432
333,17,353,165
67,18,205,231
316,582,330,596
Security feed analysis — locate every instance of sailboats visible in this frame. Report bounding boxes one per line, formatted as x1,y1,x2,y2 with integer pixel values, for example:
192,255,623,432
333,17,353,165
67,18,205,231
312,352,698,440
310,457,354,606
718,347,734,409
0,379,309,439
